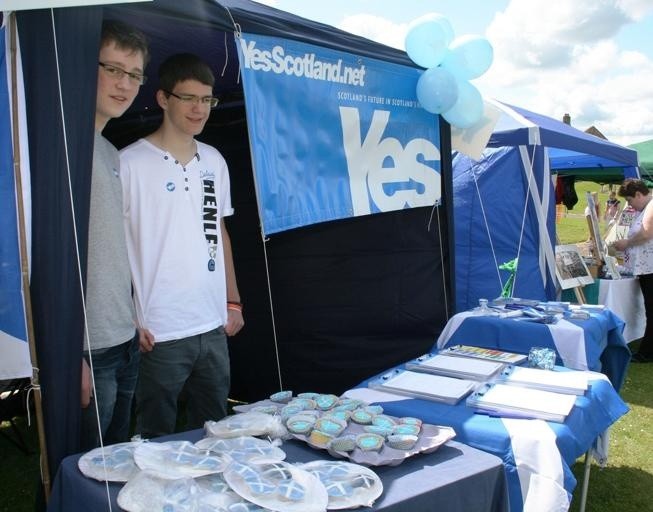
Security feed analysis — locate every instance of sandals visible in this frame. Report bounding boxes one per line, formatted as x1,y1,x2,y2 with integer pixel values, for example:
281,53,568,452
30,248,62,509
632,352,653,362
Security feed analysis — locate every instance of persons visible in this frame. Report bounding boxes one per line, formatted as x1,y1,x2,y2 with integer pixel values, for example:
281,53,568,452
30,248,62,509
604,191,621,223
612,177,653,363
77,18,142,449
592,192,601,222
118,51,248,439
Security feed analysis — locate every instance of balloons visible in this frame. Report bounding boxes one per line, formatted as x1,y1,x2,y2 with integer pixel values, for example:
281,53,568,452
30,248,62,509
403,11,495,131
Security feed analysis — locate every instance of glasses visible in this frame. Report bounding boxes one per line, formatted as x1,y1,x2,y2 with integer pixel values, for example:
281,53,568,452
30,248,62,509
99,62,145,85
162,89,219,107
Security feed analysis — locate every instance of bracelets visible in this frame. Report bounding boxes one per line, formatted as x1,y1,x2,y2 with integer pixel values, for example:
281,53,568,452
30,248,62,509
227,299,243,312
